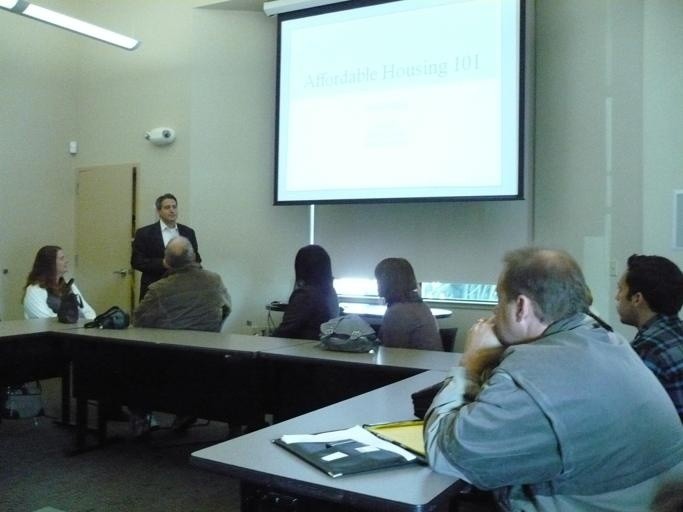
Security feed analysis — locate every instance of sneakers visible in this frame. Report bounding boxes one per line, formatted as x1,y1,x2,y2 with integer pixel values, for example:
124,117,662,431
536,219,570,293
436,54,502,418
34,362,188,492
107,409,128,422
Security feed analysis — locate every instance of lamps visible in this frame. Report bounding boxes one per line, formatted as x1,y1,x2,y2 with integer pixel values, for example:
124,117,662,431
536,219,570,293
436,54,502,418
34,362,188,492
0,0,142,52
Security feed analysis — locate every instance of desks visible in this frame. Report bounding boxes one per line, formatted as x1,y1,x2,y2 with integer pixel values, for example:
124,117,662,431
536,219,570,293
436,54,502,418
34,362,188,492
1,318,94,413
188,369,466,512
257,340,465,422
54,323,310,459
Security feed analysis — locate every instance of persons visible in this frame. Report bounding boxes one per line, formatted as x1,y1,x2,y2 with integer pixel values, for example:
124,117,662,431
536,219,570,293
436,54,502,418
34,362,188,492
131,236,232,434
21,246,130,423
615,254,683,423
375,258,445,350
131,194,201,304
272,245,340,341
423,245,683,512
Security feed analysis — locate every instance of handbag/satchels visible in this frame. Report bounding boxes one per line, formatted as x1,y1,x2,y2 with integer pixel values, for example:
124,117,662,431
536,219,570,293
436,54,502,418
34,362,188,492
411,383,444,420
58,279,79,324
84,306,130,329
318,315,380,353
